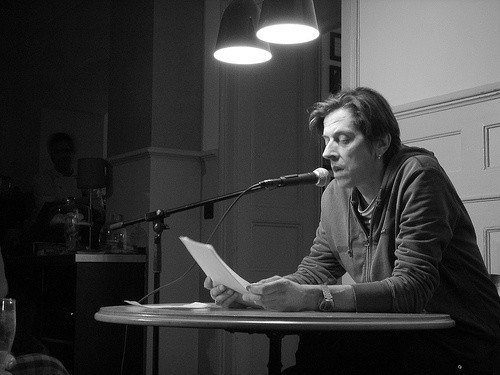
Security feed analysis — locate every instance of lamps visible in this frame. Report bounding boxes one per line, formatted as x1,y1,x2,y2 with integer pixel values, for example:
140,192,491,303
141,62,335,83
257,0,321,45
77,157,109,254
213,0,271,66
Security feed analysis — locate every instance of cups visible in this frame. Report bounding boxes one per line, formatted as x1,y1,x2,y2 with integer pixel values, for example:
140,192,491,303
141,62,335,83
0,298,16,375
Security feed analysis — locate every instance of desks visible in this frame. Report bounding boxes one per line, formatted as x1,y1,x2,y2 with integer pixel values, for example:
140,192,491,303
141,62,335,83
28,244,148,375
95,303,455,375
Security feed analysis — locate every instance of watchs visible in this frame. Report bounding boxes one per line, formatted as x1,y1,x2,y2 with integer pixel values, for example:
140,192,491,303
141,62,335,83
319,285,335,312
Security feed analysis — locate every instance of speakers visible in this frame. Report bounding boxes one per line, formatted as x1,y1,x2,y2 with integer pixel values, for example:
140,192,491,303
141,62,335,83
77,158,109,189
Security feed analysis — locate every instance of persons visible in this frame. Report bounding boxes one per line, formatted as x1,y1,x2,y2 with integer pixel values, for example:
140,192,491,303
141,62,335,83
204,87,500,375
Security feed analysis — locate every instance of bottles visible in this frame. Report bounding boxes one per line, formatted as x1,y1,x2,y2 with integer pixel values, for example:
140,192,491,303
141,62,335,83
100,214,136,254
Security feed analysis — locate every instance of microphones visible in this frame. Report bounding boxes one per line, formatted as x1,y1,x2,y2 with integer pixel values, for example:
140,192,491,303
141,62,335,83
259,168,331,188
